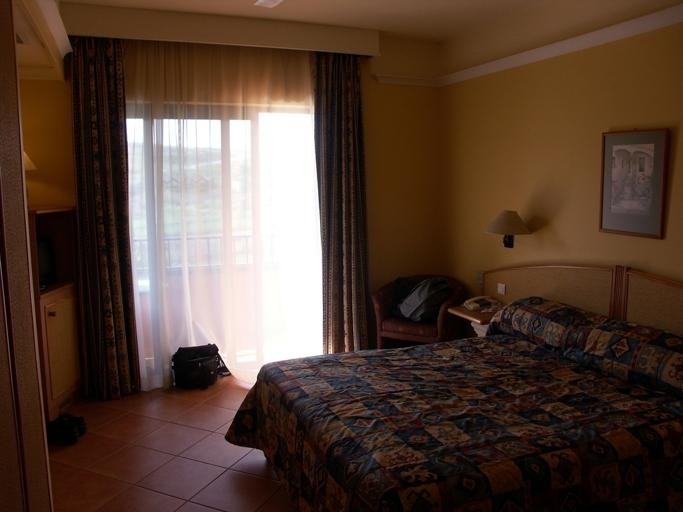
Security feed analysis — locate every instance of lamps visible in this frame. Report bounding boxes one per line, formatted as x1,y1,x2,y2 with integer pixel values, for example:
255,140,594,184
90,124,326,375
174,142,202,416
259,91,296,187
485,209,531,248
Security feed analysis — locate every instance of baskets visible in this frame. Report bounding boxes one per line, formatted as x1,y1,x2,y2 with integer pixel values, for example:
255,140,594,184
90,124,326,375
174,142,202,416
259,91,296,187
172,344,219,390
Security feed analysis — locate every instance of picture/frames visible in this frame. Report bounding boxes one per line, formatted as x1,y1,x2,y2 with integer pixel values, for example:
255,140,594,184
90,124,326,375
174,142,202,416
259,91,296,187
597,124,669,241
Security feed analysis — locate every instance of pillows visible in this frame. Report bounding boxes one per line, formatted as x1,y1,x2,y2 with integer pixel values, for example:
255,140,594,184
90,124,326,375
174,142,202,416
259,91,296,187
486,295,683,398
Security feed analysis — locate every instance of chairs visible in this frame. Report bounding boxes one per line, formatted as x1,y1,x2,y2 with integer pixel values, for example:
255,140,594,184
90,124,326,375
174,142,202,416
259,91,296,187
369,276,466,349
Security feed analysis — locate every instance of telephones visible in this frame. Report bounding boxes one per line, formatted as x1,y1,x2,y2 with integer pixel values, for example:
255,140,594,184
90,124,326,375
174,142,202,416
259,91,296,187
464,296,498,311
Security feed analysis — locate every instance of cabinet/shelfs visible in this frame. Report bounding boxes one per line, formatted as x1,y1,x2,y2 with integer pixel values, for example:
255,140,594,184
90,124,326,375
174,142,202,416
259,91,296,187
26,203,86,422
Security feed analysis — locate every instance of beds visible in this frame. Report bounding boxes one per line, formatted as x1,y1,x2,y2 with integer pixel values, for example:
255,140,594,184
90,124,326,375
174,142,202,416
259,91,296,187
254,263,683,511
